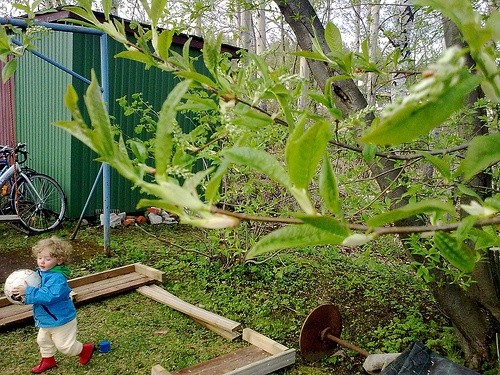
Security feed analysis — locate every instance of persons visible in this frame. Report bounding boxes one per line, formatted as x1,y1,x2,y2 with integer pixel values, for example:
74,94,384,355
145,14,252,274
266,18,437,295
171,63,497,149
11,236,94,373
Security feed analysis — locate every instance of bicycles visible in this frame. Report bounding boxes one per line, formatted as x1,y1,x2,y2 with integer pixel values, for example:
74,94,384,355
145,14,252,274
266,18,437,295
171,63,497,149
0,143,66,233
0,144,46,225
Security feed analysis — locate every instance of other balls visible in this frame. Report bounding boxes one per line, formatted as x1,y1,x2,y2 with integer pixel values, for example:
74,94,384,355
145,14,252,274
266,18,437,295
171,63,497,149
4,269,42,306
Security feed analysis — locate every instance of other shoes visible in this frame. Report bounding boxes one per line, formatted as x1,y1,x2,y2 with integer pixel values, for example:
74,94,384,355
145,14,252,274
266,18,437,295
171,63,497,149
80,345,94,364
32,357,57,373
